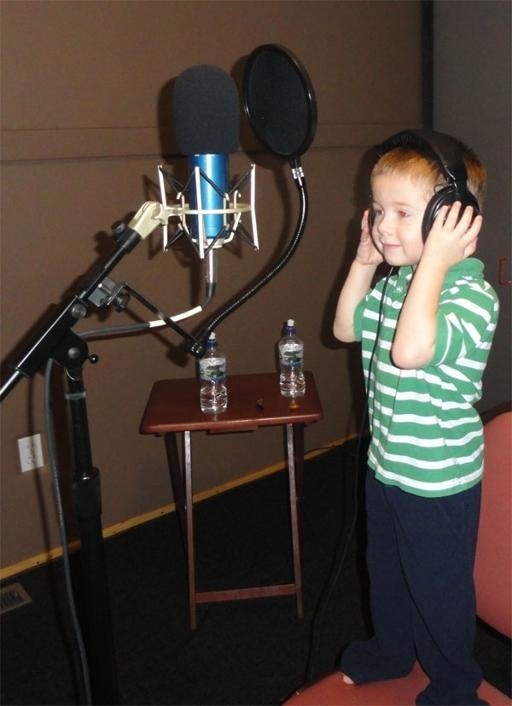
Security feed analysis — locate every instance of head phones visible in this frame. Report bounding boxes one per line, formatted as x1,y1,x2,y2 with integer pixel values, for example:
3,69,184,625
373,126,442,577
367,128,481,246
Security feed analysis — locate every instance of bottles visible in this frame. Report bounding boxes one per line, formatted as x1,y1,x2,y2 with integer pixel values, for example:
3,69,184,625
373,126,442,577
198,332,229,415
277,319,306,397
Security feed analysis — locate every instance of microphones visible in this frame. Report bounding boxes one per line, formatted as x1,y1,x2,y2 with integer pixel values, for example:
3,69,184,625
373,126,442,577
171,64,241,298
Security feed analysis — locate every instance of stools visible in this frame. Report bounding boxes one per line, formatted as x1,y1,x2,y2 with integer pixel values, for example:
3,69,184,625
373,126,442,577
139,371,325,629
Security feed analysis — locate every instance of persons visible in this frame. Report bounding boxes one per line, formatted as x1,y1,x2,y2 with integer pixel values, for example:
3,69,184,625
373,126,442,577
331,129,500,705
